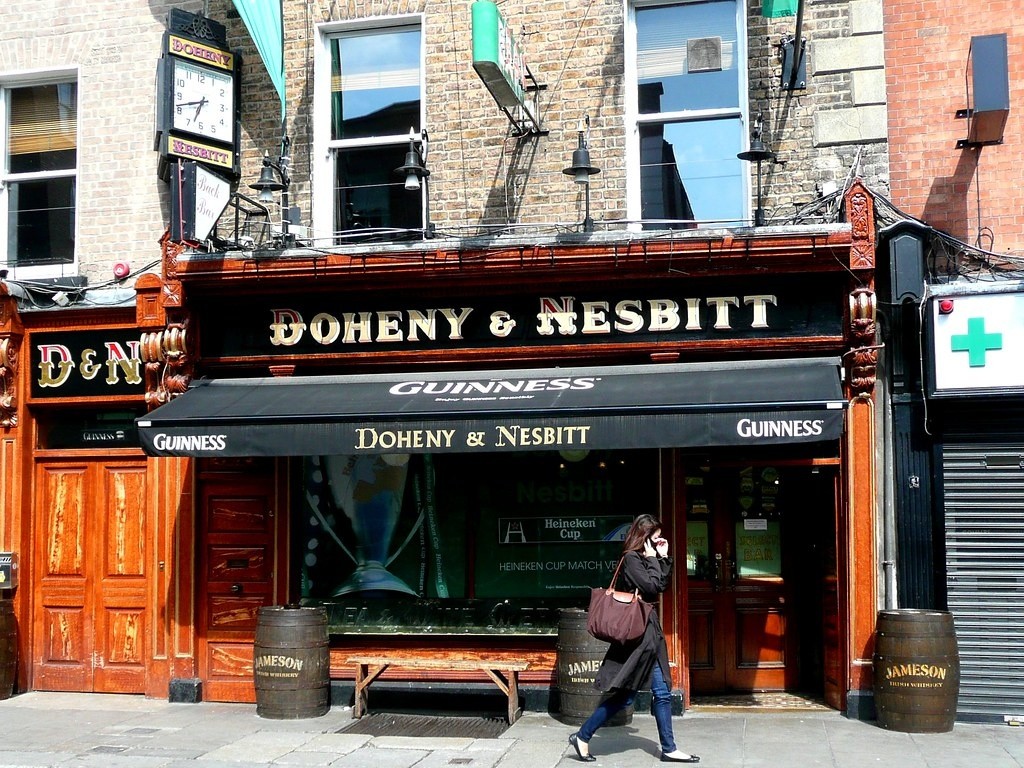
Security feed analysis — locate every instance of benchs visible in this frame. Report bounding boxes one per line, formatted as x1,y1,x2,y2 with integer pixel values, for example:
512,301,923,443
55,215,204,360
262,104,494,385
347,655,531,725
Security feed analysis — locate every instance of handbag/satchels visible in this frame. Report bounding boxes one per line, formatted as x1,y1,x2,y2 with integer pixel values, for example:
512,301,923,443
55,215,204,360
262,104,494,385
585,588,653,647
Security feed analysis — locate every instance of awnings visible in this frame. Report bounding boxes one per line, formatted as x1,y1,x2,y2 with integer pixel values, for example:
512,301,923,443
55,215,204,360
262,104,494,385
134,363,849,458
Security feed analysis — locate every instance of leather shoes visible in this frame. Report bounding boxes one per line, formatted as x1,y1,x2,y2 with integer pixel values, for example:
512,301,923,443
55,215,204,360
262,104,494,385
660,753,700,764
569,733,596,763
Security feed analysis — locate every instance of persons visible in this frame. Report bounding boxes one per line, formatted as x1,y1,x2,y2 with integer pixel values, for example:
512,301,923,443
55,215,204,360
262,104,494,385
568,514,701,763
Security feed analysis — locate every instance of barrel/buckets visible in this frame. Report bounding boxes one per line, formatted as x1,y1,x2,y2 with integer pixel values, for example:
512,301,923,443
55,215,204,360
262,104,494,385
253,604,330,721
871,608,962,731
555,618,634,727
1,598,18,700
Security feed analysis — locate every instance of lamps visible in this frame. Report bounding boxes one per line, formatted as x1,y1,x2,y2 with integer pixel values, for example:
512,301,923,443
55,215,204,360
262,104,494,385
563,116,602,232
735,110,777,227
391,125,435,240
248,148,297,249
